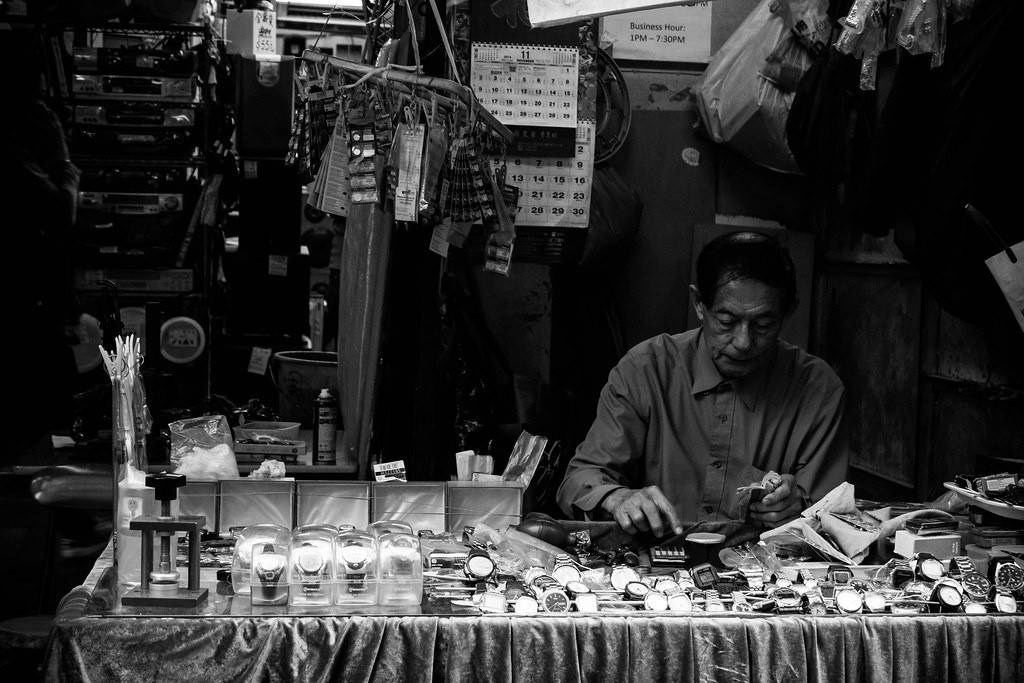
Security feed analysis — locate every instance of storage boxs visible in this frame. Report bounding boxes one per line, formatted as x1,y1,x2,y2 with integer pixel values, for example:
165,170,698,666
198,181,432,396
178,480,527,538
233,421,301,440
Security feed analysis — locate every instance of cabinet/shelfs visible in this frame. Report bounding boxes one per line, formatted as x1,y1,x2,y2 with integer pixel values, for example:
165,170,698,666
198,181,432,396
0,15,226,402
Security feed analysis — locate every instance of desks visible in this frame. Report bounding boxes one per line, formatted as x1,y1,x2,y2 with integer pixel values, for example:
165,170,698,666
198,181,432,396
43,520,1024,683
1,428,358,473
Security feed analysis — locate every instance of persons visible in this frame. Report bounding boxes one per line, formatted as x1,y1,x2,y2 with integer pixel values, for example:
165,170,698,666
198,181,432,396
554,228,854,542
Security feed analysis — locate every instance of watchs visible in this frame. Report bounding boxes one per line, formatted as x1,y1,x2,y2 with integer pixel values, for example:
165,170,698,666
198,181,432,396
385,534,418,594
252,541,288,600
293,539,330,599
416,550,1024,615
337,538,373,595
